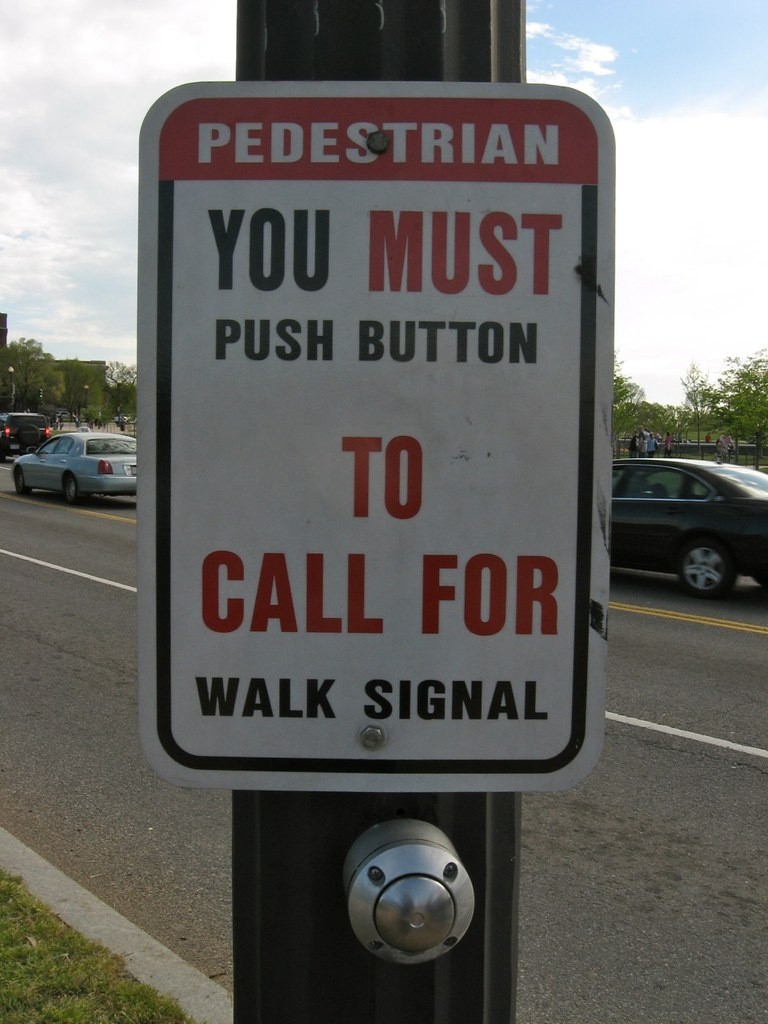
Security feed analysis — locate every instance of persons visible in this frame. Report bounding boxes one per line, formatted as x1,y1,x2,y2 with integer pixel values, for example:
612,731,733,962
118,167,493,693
716,431,736,464
705,432,712,443
628,424,672,458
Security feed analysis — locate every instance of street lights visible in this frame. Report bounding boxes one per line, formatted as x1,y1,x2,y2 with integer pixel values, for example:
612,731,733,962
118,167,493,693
83,384,90,423
8,366,15,412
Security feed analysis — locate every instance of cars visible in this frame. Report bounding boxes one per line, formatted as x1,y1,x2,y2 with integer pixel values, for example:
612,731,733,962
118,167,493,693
10,432,137,505
605,458,768,601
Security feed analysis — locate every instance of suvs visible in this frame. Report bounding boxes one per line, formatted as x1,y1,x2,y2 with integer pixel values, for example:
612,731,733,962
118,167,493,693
0,412,51,464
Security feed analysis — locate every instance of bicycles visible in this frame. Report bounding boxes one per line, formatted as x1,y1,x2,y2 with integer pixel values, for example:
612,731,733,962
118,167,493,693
712,443,738,464
